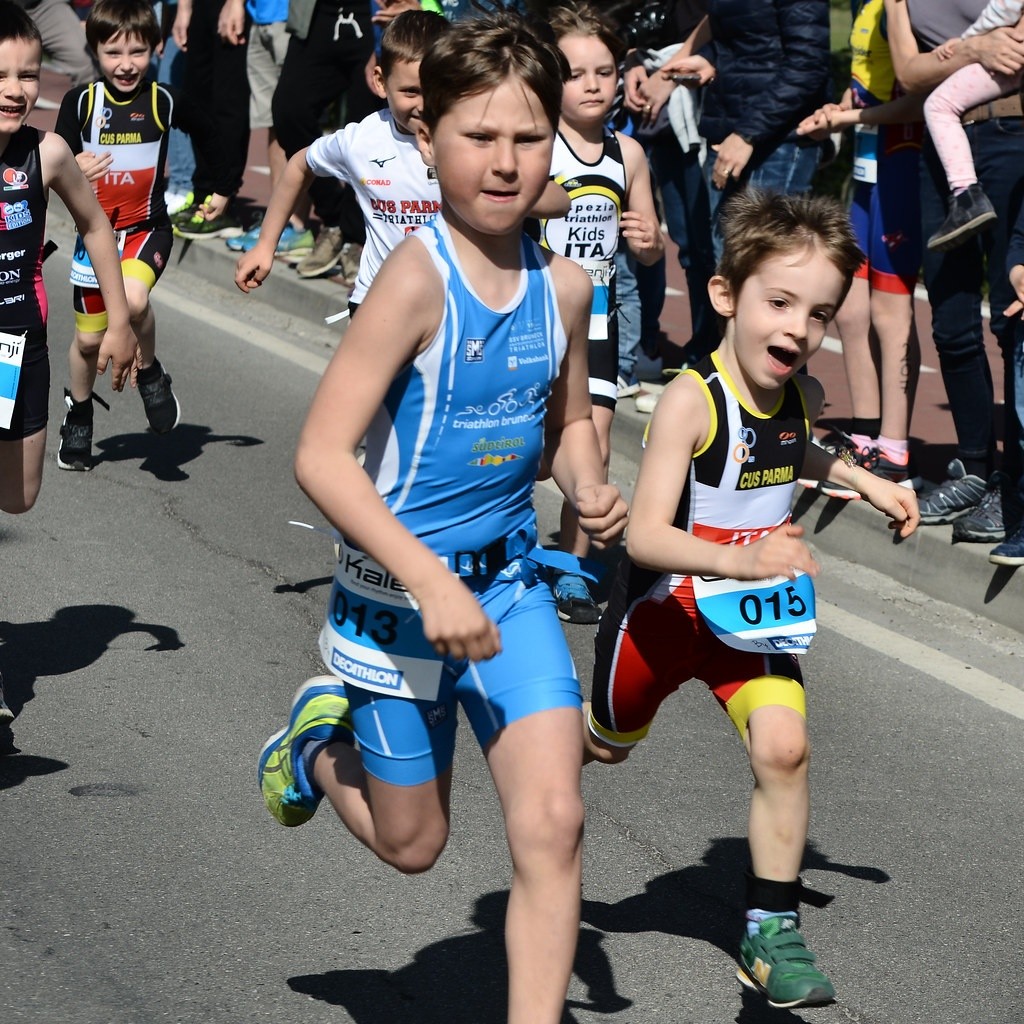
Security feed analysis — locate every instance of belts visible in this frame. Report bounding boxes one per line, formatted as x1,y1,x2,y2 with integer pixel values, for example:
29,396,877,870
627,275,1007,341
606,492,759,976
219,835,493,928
961,93,1024,124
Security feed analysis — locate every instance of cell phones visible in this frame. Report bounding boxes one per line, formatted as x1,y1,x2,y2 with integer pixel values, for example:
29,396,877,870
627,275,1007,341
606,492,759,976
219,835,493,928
670,71,701,82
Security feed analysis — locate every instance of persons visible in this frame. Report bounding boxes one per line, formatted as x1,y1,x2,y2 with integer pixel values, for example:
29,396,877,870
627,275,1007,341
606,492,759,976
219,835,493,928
255,13,630,1024
528,0,667,624
46,0,233,470
233,9,461,329
574,194,918,1010
0,5,141,515
0,0,541,292
610,0,1024,566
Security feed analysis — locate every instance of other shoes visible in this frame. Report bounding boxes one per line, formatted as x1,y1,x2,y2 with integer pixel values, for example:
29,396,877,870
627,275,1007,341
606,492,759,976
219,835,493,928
926,183,996,249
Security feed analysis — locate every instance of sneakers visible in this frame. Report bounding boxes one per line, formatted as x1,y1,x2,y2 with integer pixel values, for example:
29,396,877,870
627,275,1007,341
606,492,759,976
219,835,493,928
633,391,662,412
137,357,181,433
616,368,640,398
797,432,868,488
548,567,601,622
817,444,923,499
953,471,1006,540
989,528,1024,564
57,389,94,473
257,676,352,828
914,459,988,524
735,910,835,1008
164,192,360,287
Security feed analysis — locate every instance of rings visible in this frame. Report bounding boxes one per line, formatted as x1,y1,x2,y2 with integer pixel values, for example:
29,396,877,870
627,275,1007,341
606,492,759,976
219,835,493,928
723,169,730,176
646,103,651,109
